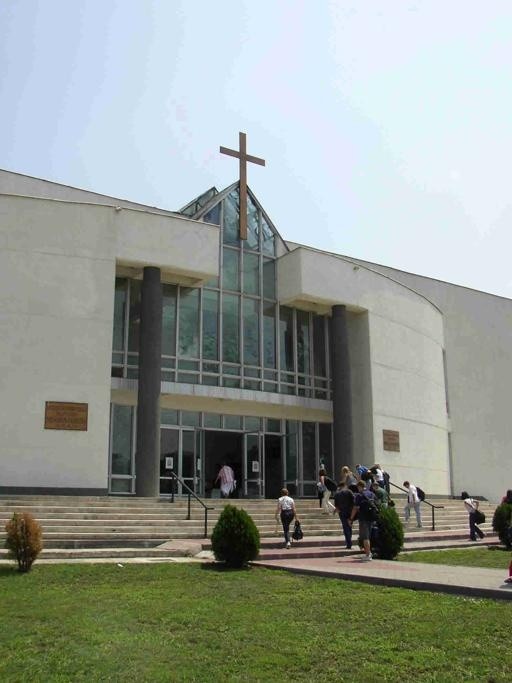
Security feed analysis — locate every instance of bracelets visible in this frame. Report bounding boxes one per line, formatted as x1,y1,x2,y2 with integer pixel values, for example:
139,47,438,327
349,518,353,521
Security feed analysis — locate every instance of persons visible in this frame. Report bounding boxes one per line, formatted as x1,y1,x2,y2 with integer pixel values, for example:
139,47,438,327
319,468,337,516
501,489,512,503
319,458,326,470
338,465,358,492
460,490,485,541
334,481,354,548
214,460,235,499
347,480,380,561
402,480,422,527
274,486,300,549
355,463,390,493
371,481,392,509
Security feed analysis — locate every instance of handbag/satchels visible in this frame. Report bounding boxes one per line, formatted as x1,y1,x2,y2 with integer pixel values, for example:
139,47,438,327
291,521,303,540
475,510,486,524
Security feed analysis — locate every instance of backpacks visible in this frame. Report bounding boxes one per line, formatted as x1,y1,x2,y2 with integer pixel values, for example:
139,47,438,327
416,486,425,501
361,491,379,523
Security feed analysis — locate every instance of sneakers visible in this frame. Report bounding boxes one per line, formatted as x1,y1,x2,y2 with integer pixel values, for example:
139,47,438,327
362,552,373,561
